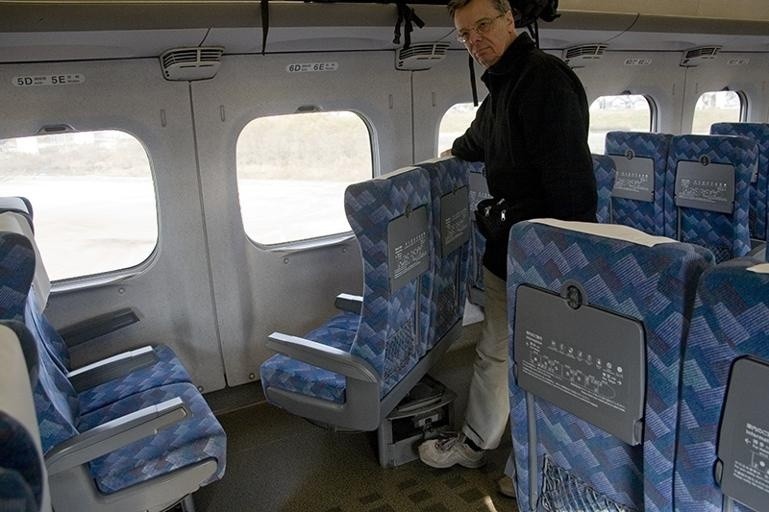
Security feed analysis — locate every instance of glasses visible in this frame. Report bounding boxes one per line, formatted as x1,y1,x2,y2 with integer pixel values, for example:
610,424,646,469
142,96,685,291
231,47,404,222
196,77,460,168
456,15,503,43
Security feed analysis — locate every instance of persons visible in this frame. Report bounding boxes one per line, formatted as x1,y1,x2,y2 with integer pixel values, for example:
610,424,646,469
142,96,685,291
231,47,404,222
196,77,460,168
416,0,599,498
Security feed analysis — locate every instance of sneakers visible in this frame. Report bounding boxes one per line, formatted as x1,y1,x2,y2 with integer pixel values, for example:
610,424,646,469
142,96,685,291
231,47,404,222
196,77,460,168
418,431,488,469
497,476,516,499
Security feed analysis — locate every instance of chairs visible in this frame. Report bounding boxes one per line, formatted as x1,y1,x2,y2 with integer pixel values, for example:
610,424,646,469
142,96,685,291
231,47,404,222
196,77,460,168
590,152,617,227
414,156,472,364
2,470,38,510
260,165,434,433
504,218,713,509
468,161,493,310
710,123,766,245
1,196,193,418
672,257,767,510
1,210,228,509
604,130,672,239
663,133,759,262
2,318,54,511
